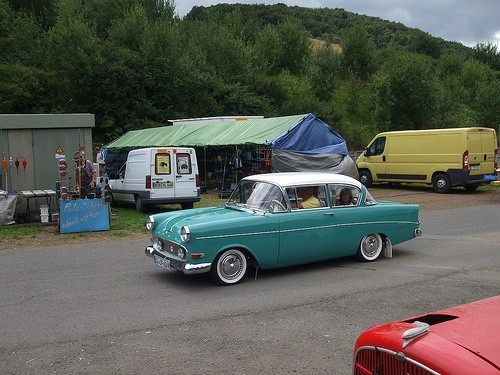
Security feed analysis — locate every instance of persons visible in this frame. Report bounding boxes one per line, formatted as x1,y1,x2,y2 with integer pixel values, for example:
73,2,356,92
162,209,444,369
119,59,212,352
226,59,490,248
291,186,354,209
74,151,95,199
95,147,104,176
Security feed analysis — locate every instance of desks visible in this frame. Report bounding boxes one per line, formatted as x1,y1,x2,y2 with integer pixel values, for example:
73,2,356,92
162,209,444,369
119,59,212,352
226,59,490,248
21,195,57,223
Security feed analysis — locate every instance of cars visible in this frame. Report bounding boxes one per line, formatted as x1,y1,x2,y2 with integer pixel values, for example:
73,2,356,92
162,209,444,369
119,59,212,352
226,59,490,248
145,171,422,286
352,293,500,375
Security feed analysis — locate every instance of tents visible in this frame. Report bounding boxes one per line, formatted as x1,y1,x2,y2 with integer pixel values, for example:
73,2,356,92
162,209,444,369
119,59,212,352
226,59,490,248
104,113,360,180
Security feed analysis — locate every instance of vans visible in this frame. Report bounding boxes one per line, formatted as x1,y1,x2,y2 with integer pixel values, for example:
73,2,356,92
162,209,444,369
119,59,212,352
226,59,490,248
354,126,500,195
105,146,202,214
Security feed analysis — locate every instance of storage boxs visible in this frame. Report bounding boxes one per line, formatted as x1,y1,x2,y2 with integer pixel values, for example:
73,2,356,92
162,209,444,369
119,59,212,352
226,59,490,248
40,208,59,222
